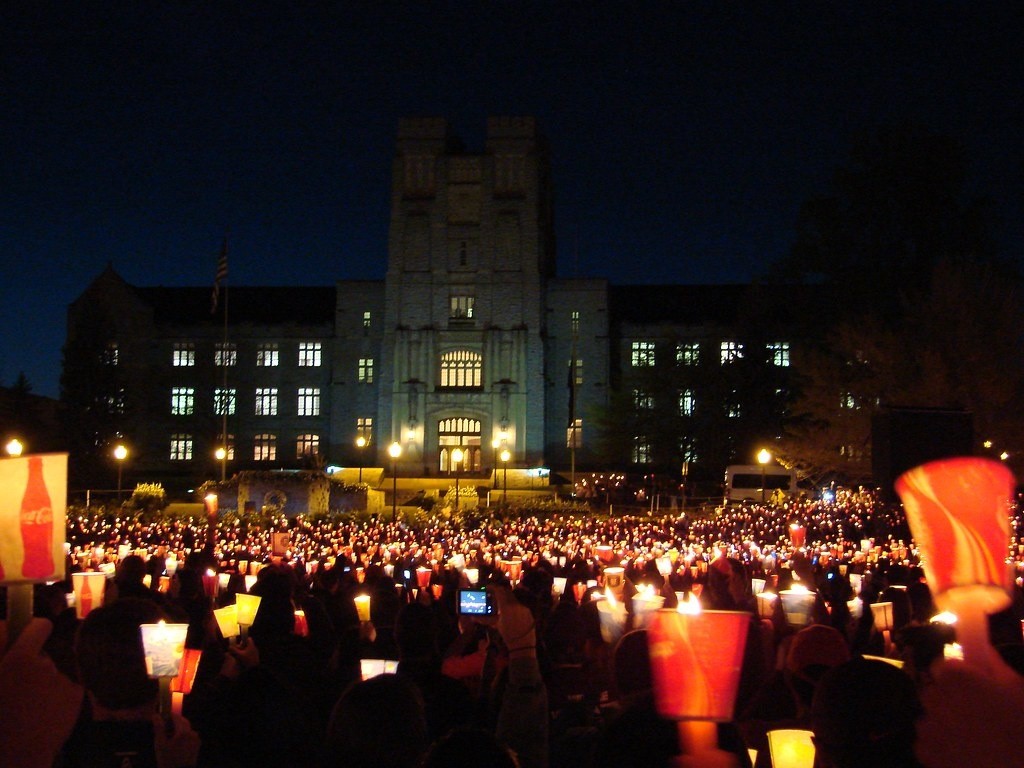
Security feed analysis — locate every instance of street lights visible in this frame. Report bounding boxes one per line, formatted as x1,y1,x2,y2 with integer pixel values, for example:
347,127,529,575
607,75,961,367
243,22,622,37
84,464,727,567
492,439,500,490
356,436,367,484
114,445,127,507
389,442,403,524
500,449,512,517
452,449,465,510
758,450,770,503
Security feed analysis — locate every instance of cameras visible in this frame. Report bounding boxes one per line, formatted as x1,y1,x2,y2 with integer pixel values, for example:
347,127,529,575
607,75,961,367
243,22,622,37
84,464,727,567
455,588,497,616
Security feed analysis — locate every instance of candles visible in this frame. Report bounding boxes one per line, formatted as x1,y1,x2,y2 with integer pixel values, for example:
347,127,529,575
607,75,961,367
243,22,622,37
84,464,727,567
894,458,1015,647
0,451,68,644
71,525,947,768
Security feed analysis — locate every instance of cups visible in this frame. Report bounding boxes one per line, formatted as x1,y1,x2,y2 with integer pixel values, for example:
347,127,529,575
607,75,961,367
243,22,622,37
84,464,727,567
139,623,189,676
213,604,240,638
76,571,105,618
0,452,69,583
235,593,262,625
271,490,1024,768
173,649,202,693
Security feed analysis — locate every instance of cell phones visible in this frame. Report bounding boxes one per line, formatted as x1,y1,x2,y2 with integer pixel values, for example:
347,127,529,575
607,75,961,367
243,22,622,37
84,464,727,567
812,554,818,565
403,569,411,587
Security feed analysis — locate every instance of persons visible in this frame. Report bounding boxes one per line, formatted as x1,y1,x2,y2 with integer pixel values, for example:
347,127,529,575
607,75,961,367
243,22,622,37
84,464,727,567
1,479,1023,767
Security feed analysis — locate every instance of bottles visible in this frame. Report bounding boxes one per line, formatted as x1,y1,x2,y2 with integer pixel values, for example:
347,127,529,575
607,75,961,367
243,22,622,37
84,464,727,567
80,575,90,618
21,458,54,579
